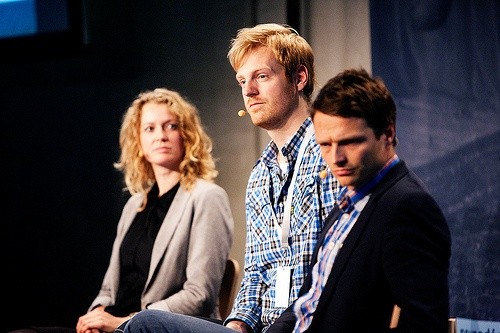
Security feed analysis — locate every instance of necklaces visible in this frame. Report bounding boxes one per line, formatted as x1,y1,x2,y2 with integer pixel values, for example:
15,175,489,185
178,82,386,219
320,157,400,248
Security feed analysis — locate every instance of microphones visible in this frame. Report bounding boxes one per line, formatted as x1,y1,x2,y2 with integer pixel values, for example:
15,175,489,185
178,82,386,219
319,166,329,179
139,151,143,157
238,110,247,116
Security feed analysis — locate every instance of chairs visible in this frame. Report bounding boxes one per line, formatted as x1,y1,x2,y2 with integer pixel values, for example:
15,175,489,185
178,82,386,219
219,257,241,321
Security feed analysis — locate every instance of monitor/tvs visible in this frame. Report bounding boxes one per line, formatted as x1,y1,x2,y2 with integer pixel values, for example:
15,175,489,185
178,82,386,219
0,0,91,54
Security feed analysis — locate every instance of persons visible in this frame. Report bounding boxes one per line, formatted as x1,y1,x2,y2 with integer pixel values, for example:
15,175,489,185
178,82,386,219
222,21,350,333
76,85,235,333
120,66,453,333
